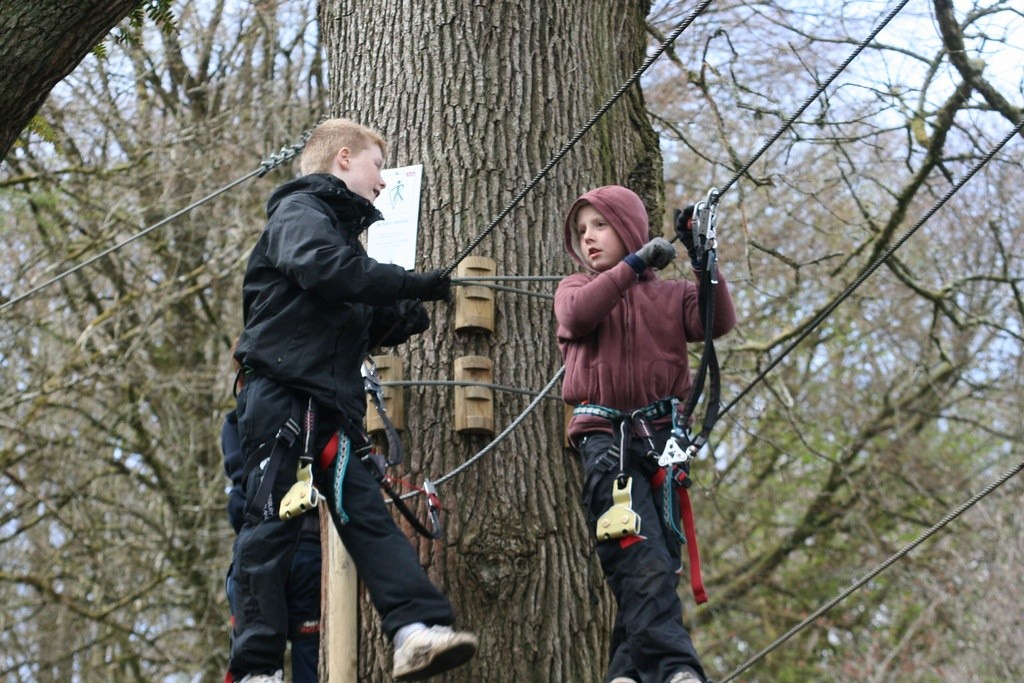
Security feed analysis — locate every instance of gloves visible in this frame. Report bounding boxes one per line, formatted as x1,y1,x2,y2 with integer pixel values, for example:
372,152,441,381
396,299,430,335
635,237,677,270
673,205,718,263
398,269,451,303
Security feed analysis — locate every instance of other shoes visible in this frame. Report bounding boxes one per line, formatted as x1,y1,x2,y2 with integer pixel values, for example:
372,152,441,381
670,672,702,683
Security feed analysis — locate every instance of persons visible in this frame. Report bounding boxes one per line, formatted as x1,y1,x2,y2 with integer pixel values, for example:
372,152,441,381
220,118,446,683
220,408,322,683
554,184,736,683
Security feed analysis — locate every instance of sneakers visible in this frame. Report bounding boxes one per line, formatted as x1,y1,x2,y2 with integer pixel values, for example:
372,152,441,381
237,670,284,683
391,624,479,681
